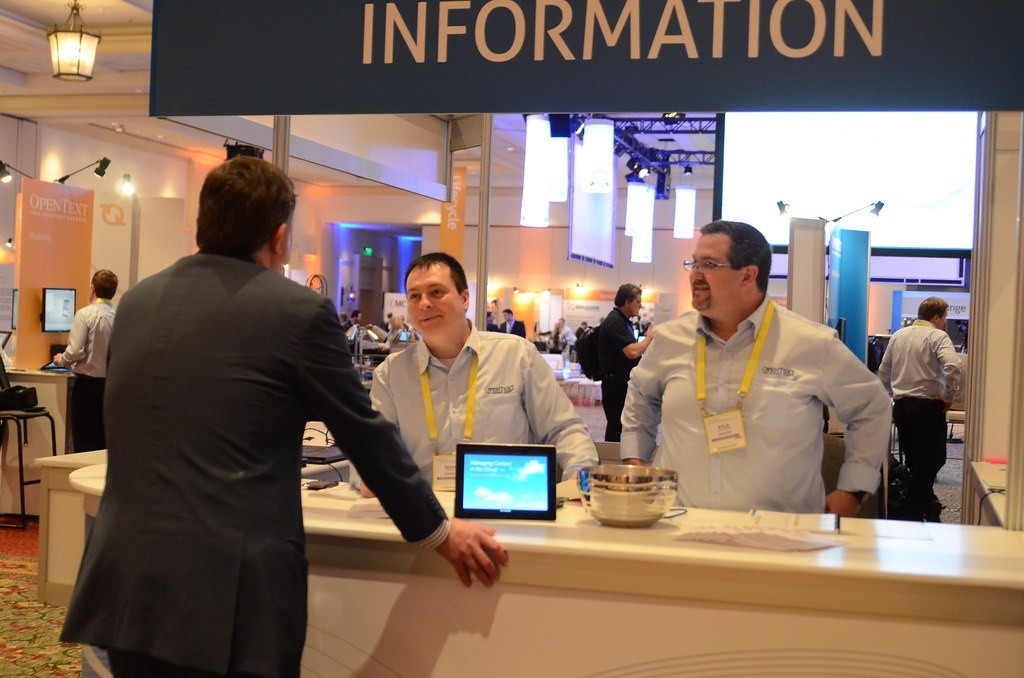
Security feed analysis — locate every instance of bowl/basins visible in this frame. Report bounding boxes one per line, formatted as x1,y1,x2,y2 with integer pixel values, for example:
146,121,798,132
576,464,679,527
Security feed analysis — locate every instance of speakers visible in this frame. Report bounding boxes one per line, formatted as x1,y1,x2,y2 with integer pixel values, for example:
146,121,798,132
549,113,572,137
655,172,671,199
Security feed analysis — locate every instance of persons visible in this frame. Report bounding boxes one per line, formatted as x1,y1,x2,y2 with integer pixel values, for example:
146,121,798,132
547,319,604,361
600,284,653,442
381,311,423,350
878,298,962,523
487,309,526,338
60,156,508,677
620,220,893,516
339,309,361,332
489,300,497,322
54,270,119,453
349,253,600,498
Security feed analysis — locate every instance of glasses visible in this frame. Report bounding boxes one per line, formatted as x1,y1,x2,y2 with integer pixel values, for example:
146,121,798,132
683,259,735,273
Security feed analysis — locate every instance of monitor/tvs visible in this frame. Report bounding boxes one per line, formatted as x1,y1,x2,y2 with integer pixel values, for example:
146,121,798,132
42,288,76,333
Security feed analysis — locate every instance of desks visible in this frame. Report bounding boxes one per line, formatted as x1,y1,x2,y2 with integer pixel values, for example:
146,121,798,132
33,446,107,607
0,369,76,518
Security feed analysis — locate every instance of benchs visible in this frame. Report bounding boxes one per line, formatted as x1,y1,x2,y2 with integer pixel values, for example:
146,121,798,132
0,410,56,530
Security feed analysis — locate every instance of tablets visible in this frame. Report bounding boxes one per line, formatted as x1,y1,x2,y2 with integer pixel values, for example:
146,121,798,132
453,443,557,522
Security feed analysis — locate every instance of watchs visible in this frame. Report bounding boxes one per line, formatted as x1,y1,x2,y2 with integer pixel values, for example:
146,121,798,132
854,490,872,505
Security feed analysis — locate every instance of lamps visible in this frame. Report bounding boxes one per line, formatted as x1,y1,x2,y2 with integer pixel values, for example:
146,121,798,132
513,287,518,294
548,114,585,140
6,238,12,247
626,158,649,180
833,201,885,223
341,285,357,306
47,0,102,82
777,201,830,223
684,165,692,175
345,324,389,343
0,160,34,184
54,157,111,184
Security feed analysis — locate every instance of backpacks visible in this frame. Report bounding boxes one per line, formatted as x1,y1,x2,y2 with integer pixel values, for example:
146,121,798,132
575,321,602,381
